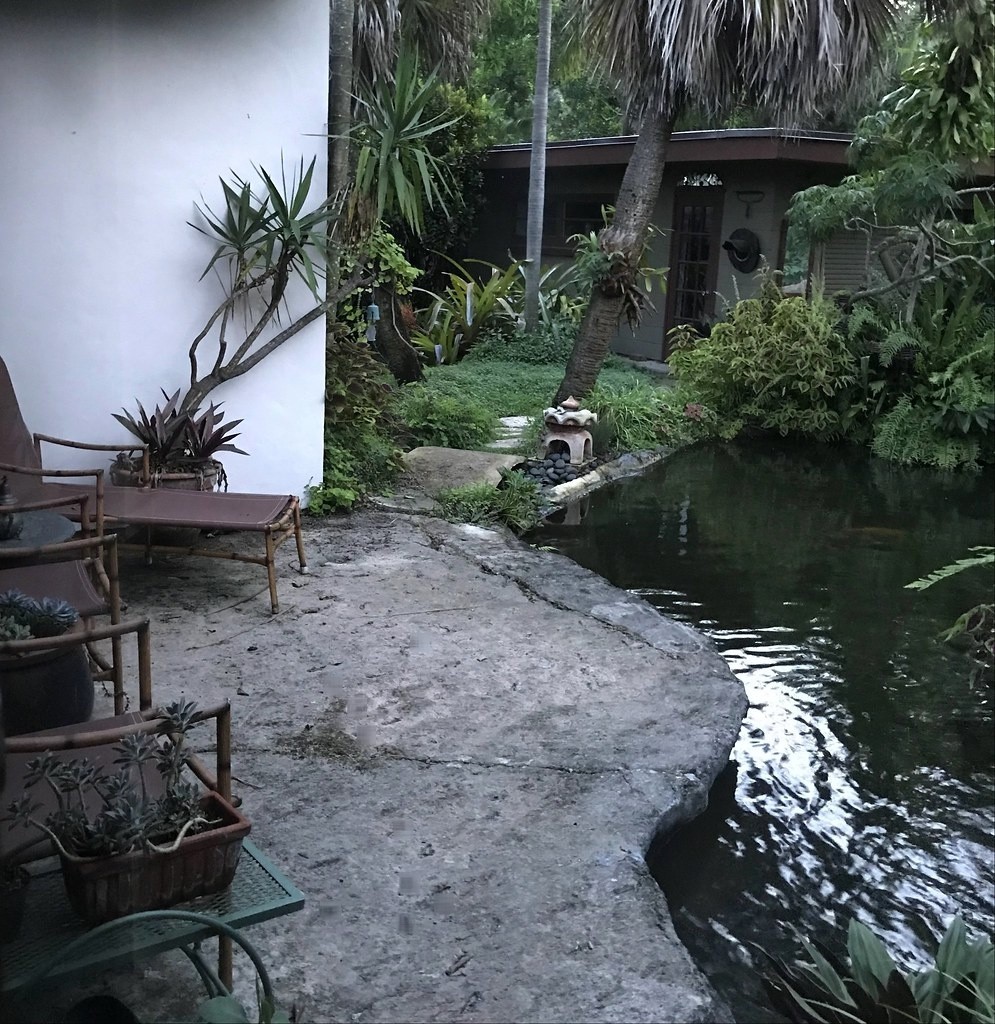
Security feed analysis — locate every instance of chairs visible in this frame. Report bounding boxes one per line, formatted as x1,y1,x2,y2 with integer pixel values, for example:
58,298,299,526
0,618,244,997
0,494,127,716
0,356,308,615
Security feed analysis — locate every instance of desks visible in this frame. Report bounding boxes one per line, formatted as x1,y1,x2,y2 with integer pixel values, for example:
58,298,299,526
0,836,306,995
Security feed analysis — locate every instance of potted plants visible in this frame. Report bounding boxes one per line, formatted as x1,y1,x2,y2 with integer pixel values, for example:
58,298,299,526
0,475,76,548
109,387,249,548
0,588,94,738
0,695,252,920
